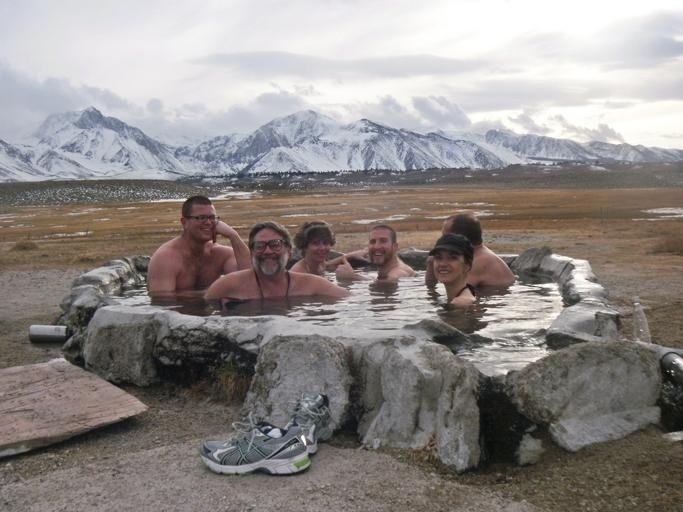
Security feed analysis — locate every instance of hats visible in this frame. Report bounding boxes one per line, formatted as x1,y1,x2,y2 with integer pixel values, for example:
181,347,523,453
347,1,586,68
429,233,473,261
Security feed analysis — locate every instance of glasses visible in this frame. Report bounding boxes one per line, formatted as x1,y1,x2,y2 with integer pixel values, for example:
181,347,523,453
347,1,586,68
183,215,220,222
250,239,289,251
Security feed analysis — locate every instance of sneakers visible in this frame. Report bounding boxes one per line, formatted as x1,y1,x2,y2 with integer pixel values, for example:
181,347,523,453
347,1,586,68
284,394,334,456
200,413,311,476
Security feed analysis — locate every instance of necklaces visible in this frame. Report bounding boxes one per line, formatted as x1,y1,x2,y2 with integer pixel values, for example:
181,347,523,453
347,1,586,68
253,268,291,302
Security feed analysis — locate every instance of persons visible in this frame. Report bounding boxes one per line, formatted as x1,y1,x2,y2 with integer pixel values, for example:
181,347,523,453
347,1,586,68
145,193,253,298
336,224,415,280
204,223,351,303
427,234,479,311
287,218,371,278
423,212,517,285
